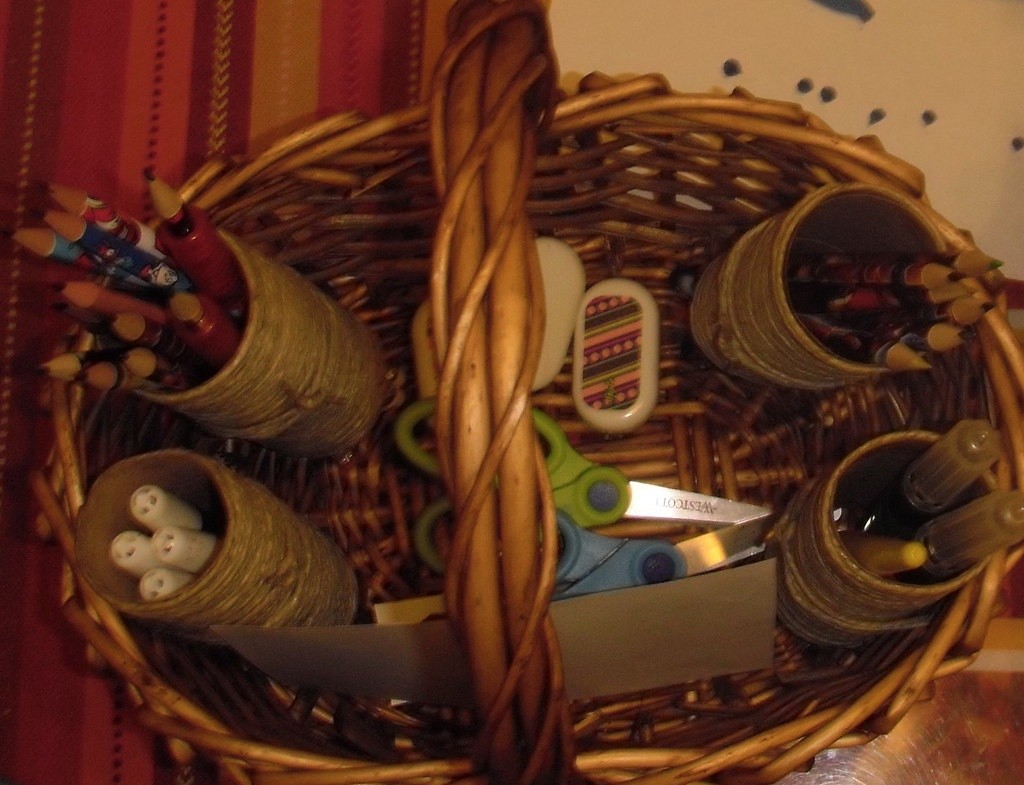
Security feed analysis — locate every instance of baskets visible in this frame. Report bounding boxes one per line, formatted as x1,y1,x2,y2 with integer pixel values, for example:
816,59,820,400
30,0,1024,783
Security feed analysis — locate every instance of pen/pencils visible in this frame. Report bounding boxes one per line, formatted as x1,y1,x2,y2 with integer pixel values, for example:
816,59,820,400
797,235,1006,372
7,176,230,397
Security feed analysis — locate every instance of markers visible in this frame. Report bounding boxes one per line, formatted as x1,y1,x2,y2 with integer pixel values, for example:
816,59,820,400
848,417,1024,582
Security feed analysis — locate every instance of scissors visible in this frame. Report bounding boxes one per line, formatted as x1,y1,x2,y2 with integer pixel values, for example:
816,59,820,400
395,369,800,635
